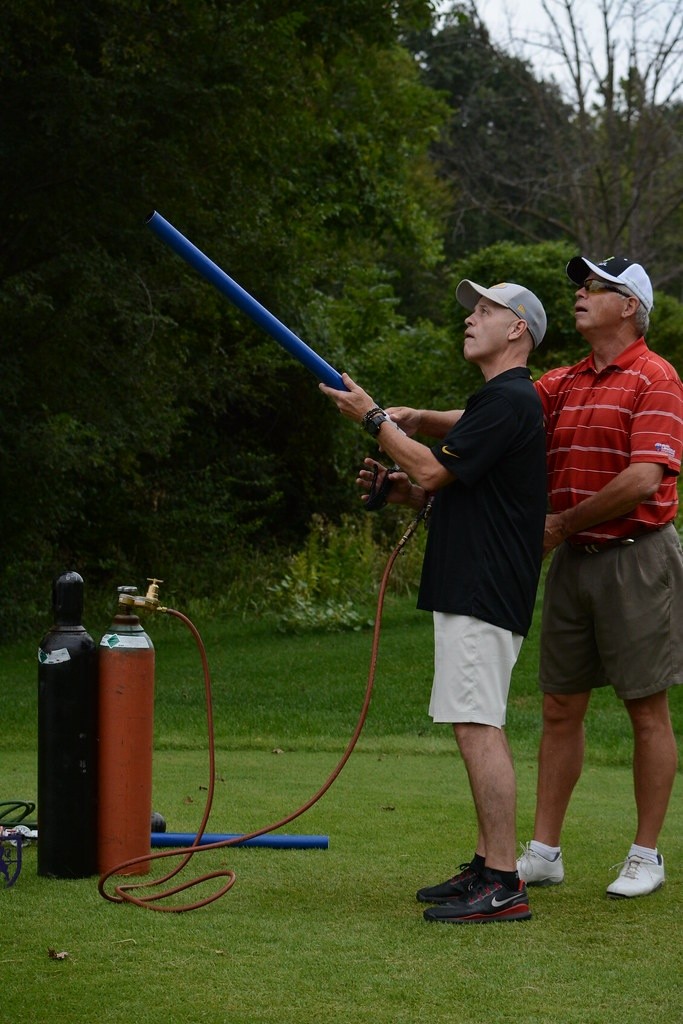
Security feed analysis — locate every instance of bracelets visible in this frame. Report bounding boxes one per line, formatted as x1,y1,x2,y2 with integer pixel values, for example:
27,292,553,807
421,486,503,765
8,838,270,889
362,408,386,431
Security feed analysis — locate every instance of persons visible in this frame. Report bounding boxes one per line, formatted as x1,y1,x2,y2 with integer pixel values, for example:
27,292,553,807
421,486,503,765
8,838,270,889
318,278,548,926
378,257,683,897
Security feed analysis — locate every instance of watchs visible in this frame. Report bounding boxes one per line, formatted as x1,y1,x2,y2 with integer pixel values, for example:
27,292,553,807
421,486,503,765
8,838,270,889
368,417,386,436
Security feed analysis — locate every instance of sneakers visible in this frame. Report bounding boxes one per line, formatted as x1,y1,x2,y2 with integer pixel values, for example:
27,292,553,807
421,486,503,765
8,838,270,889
416,861,484,904
606,853,665,898
422,871,532,923
515,840,565,886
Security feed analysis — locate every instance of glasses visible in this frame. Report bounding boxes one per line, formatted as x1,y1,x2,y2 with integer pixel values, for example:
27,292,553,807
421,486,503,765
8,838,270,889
578,279,630,298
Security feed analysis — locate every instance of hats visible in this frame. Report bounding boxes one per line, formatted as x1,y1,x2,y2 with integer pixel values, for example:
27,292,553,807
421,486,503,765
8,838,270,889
567,256,654,314
456,279,548,350
